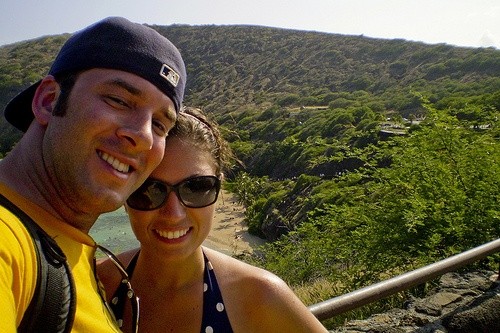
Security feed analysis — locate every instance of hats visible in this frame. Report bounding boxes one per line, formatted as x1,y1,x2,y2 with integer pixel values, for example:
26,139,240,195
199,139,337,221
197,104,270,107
3,17,187,134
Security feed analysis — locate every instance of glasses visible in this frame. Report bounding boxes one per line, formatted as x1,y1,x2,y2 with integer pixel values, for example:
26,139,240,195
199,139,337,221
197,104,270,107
125,175,221,211
93,244,141,333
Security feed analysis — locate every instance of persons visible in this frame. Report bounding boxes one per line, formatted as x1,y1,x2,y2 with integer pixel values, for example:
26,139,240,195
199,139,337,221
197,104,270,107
0,16,188,333
92,107,330,332
